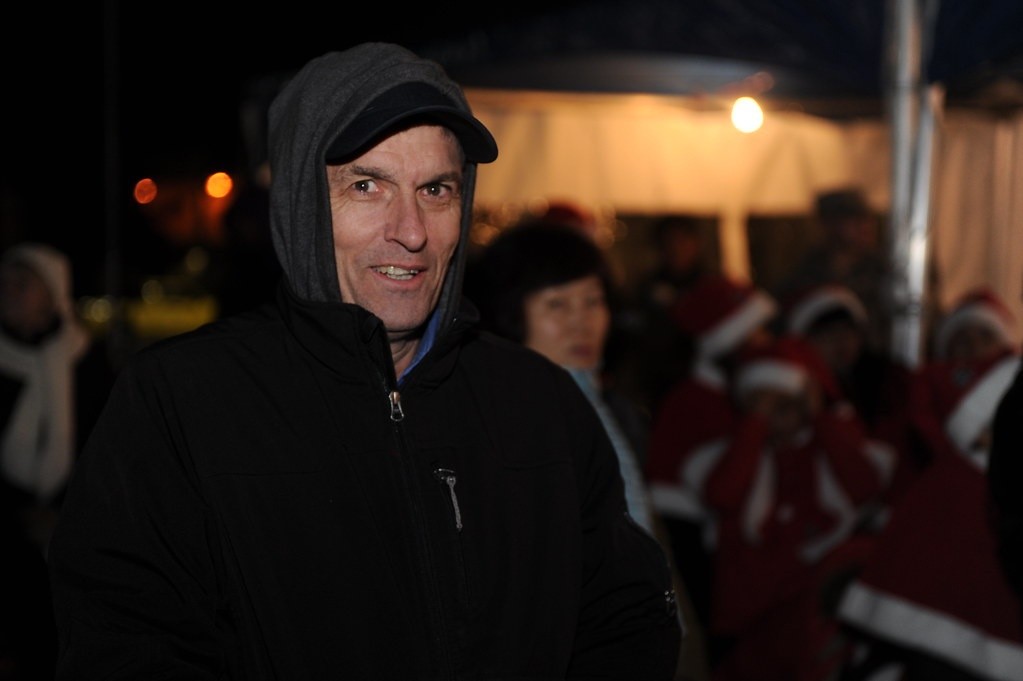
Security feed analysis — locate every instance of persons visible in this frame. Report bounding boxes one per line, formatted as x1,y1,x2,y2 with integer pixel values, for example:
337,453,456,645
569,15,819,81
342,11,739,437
607,192,1023,681
472,217,654,532
53,42,683,680
1,246,85,680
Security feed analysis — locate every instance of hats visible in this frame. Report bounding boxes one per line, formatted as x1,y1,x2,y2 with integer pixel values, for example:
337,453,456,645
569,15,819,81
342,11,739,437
786,279,869,345
741,332,853,423
5,245,72,314
678,268,777,357
922,344,1019,452
324,81,497,170
938,285,1022,372
